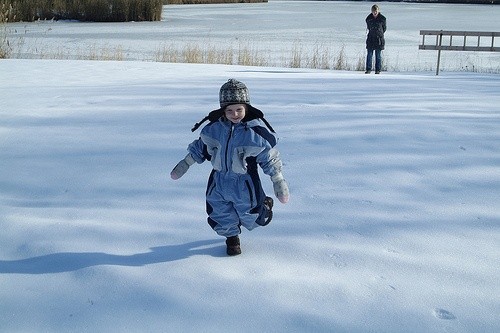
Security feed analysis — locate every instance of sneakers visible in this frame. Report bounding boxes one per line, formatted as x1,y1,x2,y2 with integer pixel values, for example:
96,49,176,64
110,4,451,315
226,236,241,255
263,197,273,225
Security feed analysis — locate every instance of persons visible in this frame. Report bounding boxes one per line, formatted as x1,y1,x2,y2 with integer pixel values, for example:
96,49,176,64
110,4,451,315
365,5,387,74
171,78,290,255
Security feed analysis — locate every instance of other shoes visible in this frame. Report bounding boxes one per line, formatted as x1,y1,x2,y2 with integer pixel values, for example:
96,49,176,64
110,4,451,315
365,70,371,74
375,71,380,74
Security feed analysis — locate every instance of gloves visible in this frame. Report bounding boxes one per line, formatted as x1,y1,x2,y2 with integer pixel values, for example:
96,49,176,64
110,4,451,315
270,171,289,204
171,154,194,180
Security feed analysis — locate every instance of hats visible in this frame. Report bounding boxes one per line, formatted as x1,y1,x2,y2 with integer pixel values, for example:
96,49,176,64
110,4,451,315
219,79,251,108
